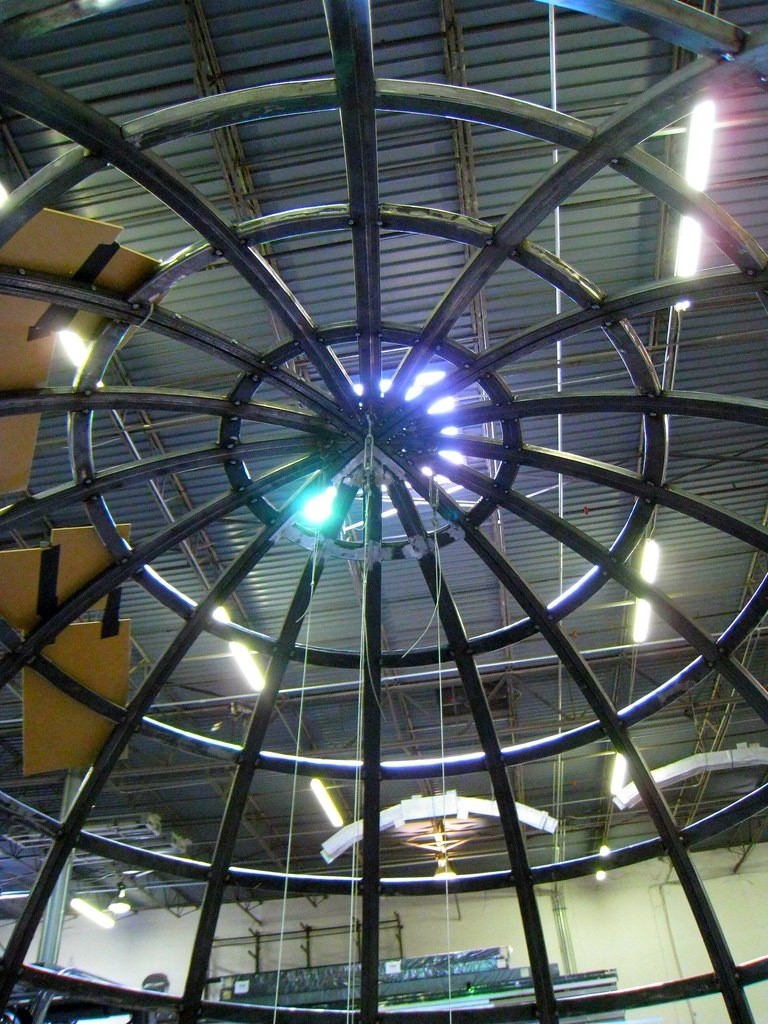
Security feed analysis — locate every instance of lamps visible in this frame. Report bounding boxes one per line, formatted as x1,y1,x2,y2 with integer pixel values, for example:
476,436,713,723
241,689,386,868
108,889,131,913
